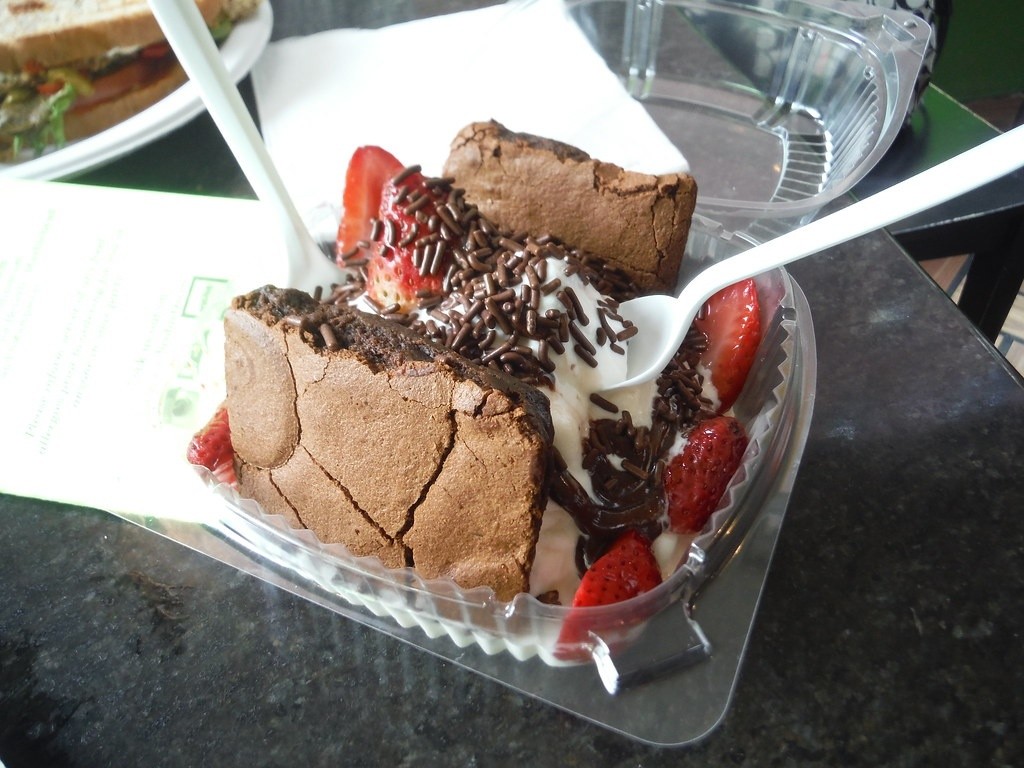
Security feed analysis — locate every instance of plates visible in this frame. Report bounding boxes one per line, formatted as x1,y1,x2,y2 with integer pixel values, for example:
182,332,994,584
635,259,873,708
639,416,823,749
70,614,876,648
1,1,271,179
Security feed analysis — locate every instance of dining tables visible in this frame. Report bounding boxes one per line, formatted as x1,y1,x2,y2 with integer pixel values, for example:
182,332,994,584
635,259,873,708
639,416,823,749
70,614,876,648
0,0,1024,768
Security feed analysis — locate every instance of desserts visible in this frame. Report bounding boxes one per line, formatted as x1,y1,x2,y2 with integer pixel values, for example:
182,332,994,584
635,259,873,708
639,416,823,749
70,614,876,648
189,119,761,668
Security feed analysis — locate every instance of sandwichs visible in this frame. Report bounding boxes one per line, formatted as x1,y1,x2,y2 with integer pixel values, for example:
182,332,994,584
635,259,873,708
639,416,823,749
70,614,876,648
0,0,266,165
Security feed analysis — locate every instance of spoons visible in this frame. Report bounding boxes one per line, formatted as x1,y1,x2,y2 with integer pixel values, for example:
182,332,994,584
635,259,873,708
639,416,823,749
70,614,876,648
604,119,1023,391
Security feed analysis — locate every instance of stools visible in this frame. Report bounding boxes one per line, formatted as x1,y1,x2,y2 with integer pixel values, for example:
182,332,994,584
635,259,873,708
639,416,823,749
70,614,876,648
837,83,1024,342
